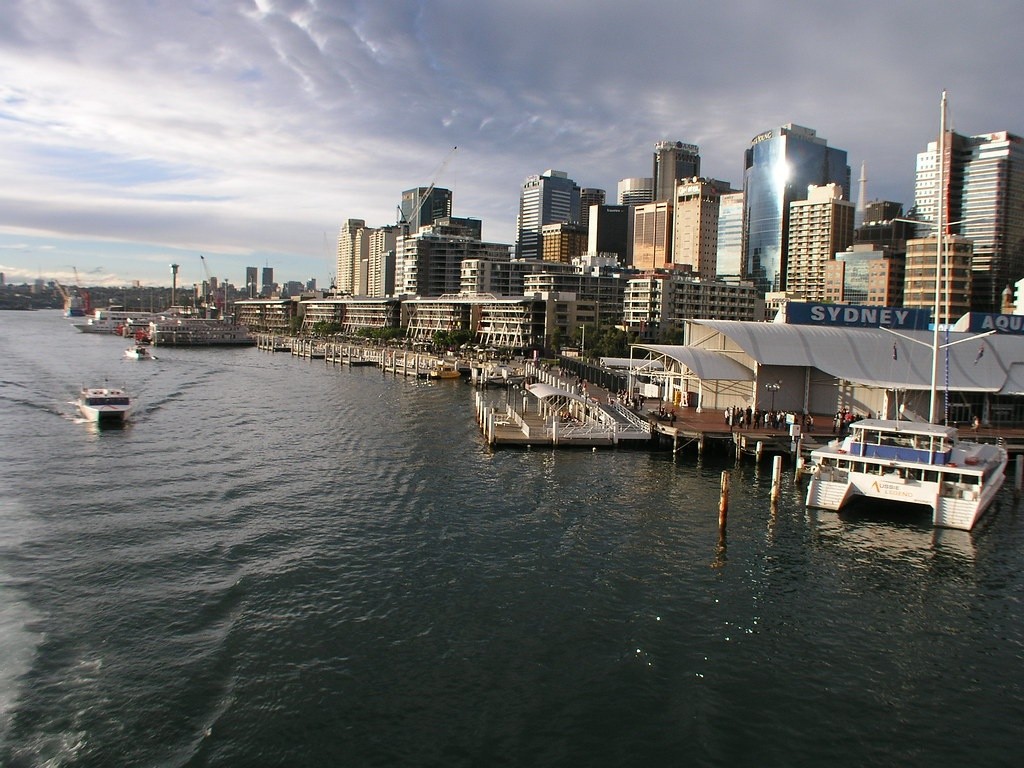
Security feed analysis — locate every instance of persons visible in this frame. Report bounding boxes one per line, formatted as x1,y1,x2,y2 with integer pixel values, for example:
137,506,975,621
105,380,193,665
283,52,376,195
616,388,645,411
813,462,822,481
831,404,880,434
724,403,816,433
970,415,979,432
568,412,571,420
831,467,837,481
558,366,587,395
534,357,548,372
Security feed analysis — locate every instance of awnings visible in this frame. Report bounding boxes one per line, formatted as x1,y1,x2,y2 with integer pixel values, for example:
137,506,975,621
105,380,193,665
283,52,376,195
598,356,664,371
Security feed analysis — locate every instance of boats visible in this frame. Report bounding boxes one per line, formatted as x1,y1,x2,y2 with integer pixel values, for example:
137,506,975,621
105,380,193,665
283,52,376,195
75,376,135,424
431,363,462,379
125,344,151,360
55,255,270,346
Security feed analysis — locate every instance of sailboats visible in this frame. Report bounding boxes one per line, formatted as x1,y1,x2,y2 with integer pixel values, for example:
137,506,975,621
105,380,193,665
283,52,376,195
804,87,1010,532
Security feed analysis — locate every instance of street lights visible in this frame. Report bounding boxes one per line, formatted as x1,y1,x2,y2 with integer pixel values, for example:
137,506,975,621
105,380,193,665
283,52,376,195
580,324,585,362
512,384,519,412
520,390,527,420
506,379,513,405
766,384,781,410
